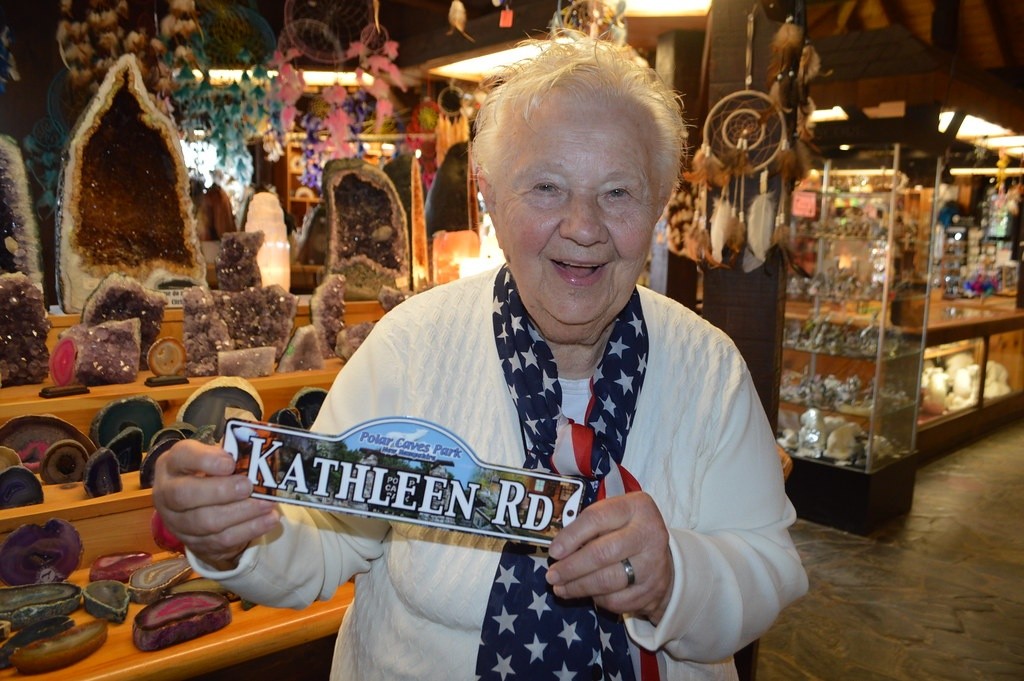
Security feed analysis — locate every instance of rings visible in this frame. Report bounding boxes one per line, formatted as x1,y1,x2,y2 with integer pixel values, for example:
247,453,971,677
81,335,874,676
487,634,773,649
620,558,636,587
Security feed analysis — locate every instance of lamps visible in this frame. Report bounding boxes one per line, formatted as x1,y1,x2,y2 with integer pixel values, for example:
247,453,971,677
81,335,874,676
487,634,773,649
192,66,377,90
587,0,713,58
420,31,578,89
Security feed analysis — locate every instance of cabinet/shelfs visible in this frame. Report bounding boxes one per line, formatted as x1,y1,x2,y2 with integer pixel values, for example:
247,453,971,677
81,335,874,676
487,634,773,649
917,281,1024,468
774,146,944,537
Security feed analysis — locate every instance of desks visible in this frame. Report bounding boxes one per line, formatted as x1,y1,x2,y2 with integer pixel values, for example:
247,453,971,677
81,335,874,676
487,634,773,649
0,286,395,681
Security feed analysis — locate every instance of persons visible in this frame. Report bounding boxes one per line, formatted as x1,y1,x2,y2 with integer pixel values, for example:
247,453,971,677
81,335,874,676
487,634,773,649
150,22,810,681
